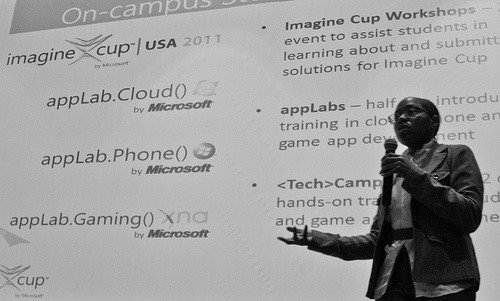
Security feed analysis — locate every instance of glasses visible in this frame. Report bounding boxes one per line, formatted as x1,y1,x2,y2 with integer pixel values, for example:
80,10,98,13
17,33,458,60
388,107,432,125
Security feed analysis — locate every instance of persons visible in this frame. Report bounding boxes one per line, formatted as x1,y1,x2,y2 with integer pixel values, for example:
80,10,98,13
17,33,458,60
277,97,484,301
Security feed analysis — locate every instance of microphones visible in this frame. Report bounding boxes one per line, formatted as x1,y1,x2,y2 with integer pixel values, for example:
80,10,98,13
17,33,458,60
382,139,398,207
